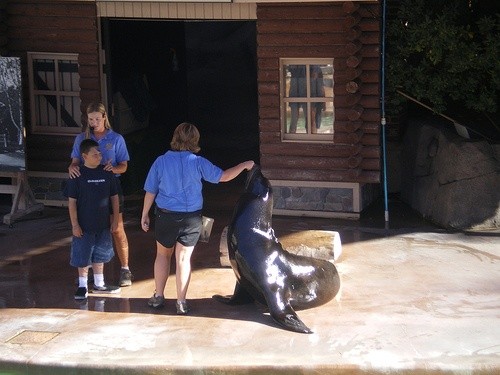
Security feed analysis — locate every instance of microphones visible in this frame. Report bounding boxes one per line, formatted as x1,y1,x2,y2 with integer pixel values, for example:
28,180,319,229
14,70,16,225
89,127,94,130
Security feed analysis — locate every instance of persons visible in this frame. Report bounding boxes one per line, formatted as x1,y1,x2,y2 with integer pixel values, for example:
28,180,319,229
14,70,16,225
66,138,122,300
141,122,255,315
286,64,327,134
68,101,132,287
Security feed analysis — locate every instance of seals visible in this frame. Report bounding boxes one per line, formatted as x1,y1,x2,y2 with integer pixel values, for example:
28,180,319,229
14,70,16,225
212,164,340,334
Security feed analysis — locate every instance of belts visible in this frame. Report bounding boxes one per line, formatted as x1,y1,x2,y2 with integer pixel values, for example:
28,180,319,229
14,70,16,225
158,209,202,215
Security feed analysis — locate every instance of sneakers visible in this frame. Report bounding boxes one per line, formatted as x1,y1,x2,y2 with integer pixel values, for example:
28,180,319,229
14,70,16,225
75,274,94,284
118,268,132,286
146,295,165,307
93,285,120,293
74,284,89,299
176,302,192,313
